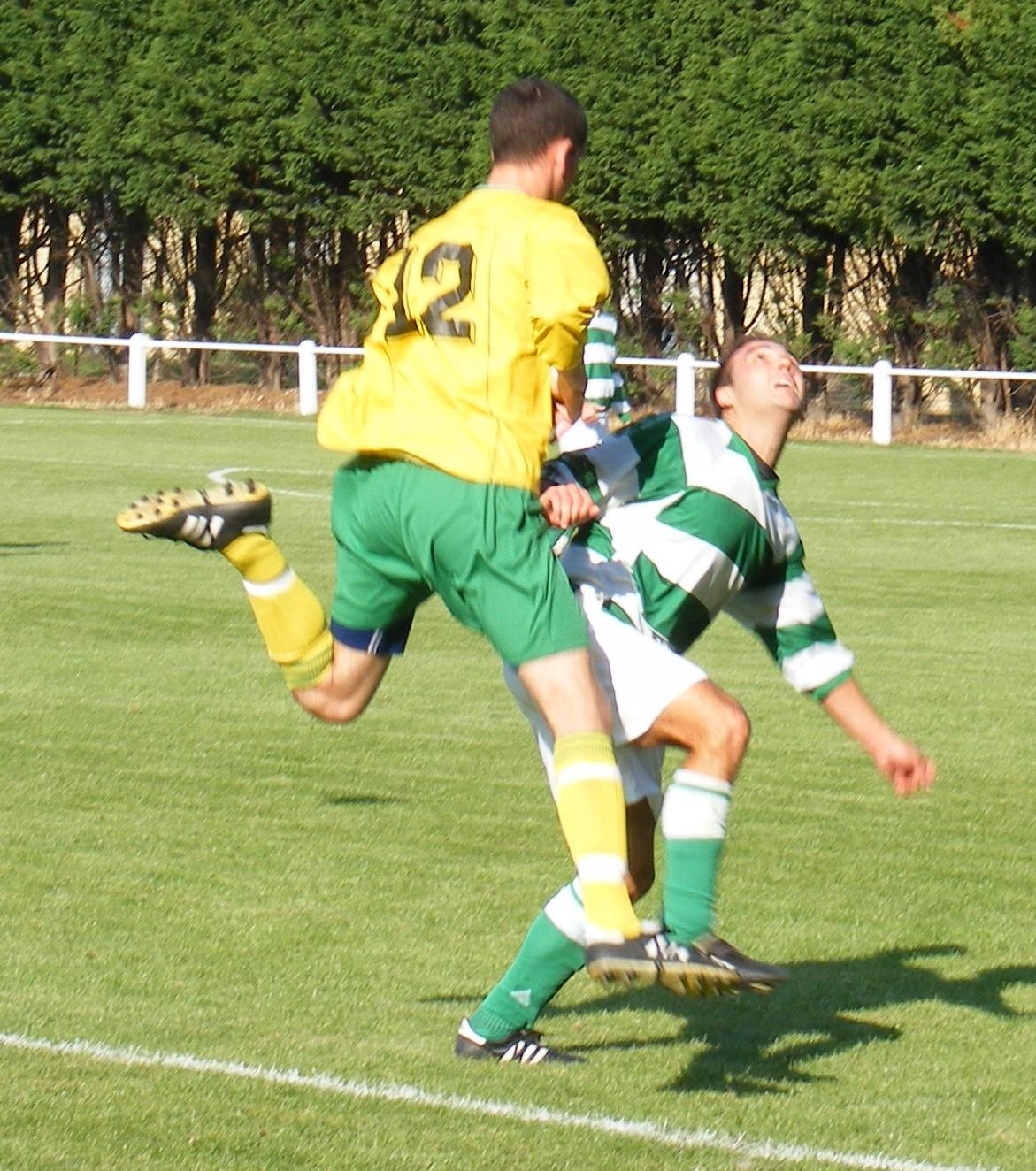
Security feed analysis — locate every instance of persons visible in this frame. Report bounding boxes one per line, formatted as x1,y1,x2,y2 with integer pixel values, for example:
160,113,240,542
453,335,938,1068
115,77,745,1003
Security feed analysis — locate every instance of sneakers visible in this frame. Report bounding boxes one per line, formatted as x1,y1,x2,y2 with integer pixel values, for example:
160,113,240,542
455,1018,587,1064
583,919,739,997
115,478,272,551
692,931,791,994
